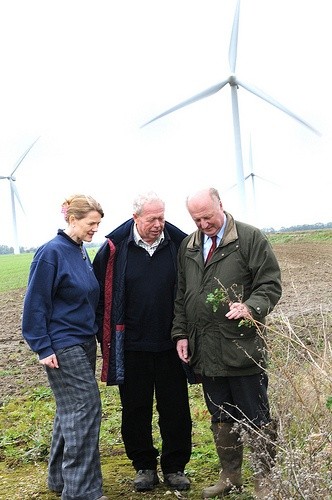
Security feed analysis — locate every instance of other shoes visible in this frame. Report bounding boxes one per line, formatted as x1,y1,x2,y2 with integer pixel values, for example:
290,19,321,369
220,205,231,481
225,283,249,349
97,496,108,500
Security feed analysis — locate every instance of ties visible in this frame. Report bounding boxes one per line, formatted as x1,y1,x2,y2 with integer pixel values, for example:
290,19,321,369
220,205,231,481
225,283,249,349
204,235,218,268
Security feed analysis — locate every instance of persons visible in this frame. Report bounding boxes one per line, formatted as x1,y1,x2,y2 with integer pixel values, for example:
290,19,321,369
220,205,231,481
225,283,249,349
21,194,109,500
92,190,192,490
171,187,282,500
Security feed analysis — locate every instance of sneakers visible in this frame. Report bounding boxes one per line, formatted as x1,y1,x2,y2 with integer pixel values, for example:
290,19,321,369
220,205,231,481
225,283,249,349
134,469,159,490
164,471,190,490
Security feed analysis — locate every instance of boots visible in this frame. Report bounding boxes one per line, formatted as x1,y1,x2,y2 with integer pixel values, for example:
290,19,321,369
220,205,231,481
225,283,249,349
202,423,244,498
248,427,278,500
